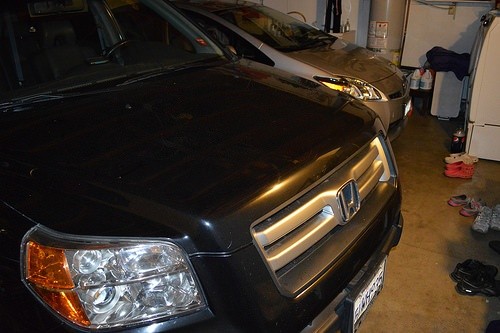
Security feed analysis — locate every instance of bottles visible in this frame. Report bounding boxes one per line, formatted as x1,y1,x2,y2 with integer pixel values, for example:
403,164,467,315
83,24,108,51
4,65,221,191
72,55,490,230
410,69,420,89
450,124,465,154
420,70,433,90
344,18,350,33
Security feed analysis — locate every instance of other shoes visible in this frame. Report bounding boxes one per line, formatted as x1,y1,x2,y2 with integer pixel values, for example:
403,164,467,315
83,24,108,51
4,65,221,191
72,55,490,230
459,201,486,217
489,240,500,254
456,271,500,297
451,259,498,283
448,194,480,207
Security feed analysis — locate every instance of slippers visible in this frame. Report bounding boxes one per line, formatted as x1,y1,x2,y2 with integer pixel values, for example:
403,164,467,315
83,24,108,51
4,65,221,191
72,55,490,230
449,152,479,162
445,161,474,169
445,156,472,164
444,167,474,178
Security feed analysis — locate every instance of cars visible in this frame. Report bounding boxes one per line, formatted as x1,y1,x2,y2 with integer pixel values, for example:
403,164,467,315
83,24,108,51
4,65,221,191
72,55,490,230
0,1,407,333
174,0,414,135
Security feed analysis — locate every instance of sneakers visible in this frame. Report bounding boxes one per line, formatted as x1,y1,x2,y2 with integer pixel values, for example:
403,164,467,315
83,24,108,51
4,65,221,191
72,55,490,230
491,204,500,231
471,206,493,234
